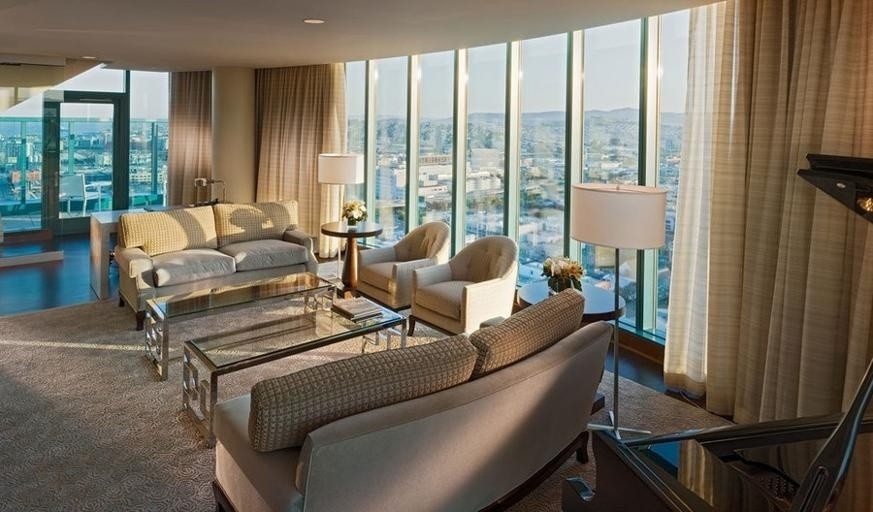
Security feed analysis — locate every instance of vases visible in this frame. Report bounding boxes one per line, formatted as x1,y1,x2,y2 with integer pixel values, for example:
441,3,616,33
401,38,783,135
547,276,572,298
348,220,357,226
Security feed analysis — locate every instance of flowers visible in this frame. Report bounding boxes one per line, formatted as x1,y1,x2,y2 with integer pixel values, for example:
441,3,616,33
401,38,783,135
340,198,369,222
541,256,583,292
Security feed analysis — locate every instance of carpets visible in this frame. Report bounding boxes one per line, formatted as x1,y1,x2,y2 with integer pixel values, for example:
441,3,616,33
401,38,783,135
0,299,732,512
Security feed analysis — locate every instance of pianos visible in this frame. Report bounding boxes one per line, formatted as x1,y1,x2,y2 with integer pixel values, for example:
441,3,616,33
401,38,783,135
561,153,869,510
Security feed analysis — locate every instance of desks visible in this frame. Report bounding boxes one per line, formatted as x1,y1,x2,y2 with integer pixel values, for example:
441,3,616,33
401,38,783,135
320,221,384,298
517,279,626,416
90,205,196,299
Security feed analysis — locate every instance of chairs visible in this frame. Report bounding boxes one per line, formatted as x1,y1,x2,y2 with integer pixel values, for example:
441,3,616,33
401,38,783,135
410,235,518,339
357,221,451,313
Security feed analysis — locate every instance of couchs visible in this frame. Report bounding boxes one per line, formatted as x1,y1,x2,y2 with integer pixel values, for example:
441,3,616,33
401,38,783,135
114,199,319,332
215,318,612,512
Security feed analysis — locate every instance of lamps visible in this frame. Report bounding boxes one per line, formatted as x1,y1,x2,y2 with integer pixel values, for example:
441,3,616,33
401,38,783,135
317,153,364,277
568,183,668,442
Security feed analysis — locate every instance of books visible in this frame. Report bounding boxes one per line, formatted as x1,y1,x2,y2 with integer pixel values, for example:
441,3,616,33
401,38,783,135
330,296,382,324
